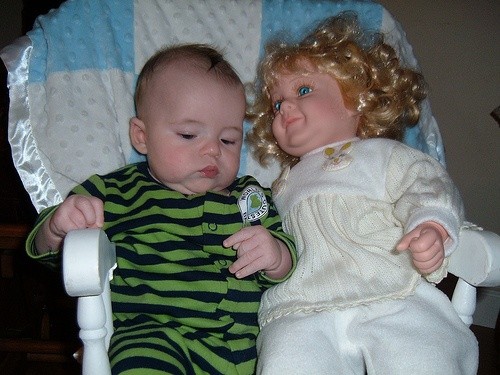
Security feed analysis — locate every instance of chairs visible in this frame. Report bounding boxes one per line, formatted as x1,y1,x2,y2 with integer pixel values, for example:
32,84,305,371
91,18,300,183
0,0,500,375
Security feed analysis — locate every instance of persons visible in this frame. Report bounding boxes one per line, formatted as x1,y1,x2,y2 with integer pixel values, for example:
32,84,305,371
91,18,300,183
24,43,298,375
243,7,480,375
